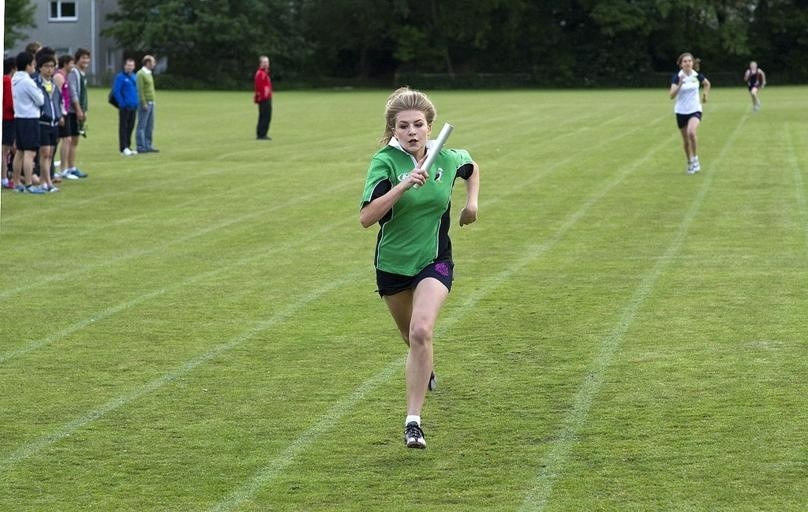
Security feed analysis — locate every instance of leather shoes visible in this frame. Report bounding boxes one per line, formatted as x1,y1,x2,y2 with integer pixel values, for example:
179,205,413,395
148,148,160,153
137,149,148,151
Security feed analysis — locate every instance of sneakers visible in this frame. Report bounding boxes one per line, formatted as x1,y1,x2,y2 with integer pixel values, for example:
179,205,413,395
60,173,70,178
688,160,696,176
67,175,79,180
47,186,58,193
692,157,702,172
119,148,132,157
54,173,59,177
428,371,438,392
32,178,40,185
13,184,27,193
257,135,271,141
52,179,63,184
131,149,139,154
2,179,12,187
405,422,427,449
26,184,43,195
68,168,87,178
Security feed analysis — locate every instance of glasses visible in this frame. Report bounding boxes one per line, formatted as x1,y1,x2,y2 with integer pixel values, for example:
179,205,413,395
41,63,57,69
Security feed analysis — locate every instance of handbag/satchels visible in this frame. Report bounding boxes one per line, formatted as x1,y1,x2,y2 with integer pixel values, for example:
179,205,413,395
108,72,136,110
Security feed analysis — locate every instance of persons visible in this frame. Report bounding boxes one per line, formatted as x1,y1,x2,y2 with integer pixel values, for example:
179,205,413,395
24,41,42,78
135,54,160,153
10,51,45,193
1,57,20,190
358,85,480,451
111,57,137,157
743,61,766,112
31,55,65,193
52,54,78,179
30,46,63,184
669,52,711,175
67,47,91,178
253,55,274,141
